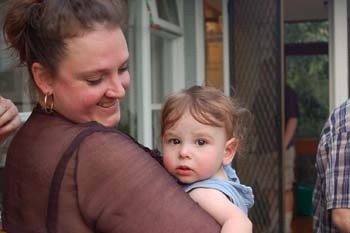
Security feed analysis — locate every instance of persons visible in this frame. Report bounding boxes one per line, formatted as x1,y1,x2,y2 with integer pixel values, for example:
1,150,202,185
312,100,350,233
0,95,21,144
156,86,254,233
249,39,299,233
2,0,222,233
